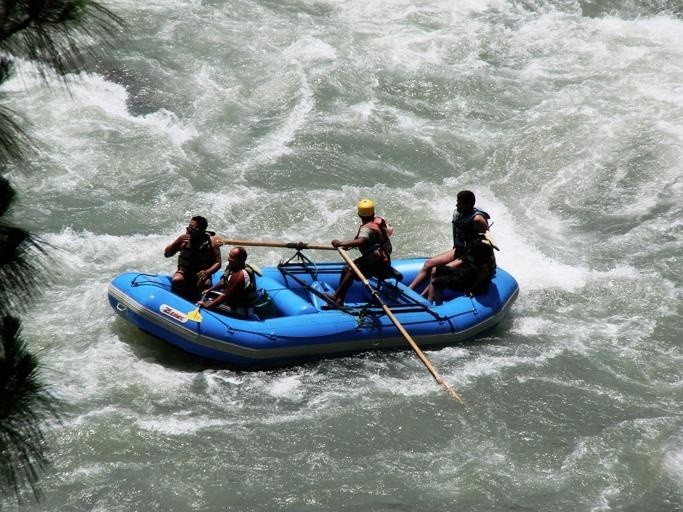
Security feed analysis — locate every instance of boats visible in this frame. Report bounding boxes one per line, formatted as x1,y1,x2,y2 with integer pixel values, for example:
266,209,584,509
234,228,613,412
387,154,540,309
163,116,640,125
108,240,520,371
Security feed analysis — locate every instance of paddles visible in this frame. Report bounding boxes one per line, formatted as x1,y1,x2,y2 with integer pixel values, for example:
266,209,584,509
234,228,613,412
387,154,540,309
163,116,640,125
337,244,464,408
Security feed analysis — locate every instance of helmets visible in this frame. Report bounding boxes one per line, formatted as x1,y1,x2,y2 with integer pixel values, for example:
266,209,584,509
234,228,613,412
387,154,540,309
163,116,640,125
357,199,375,217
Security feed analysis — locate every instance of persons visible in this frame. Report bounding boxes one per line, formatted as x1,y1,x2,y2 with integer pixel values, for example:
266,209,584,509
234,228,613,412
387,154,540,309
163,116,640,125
409,190,499,304
321,200,394,312
164,216,262,319
